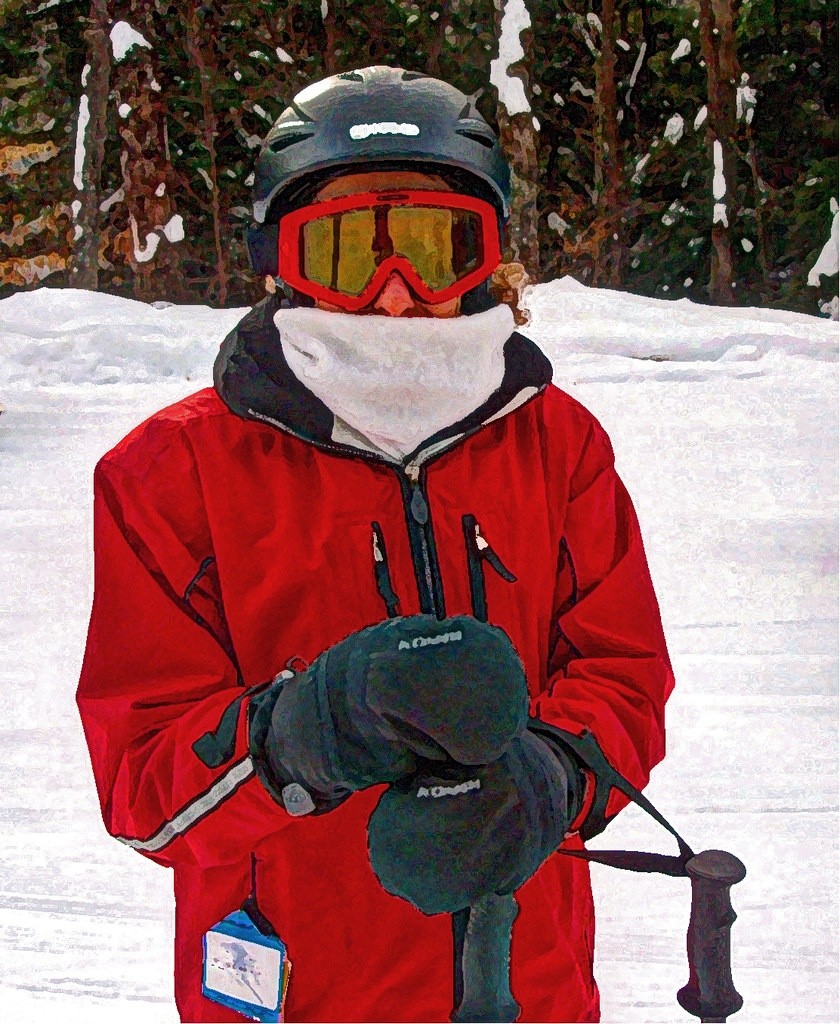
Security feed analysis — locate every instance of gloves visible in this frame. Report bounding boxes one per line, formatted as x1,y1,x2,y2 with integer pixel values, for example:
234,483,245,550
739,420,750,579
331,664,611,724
364,724,580,915
259,612,529,814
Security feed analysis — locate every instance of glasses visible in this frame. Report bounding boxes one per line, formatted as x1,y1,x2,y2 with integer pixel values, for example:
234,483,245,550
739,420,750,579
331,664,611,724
275,188,500,312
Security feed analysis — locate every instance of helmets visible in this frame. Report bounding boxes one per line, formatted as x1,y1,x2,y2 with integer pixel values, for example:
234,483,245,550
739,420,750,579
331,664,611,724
252,63,508,224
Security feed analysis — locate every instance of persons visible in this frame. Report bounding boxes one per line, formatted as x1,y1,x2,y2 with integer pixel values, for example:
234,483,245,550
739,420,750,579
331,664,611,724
76,65,677,1023
